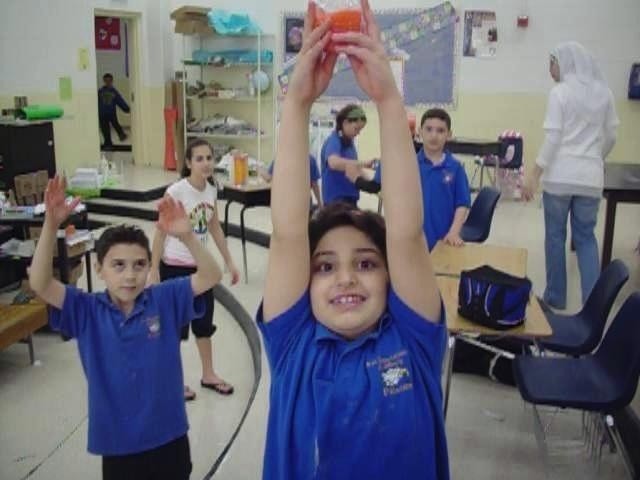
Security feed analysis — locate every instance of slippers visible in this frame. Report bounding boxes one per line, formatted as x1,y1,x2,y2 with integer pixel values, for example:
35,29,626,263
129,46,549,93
199,379,234,395
183,385,197,401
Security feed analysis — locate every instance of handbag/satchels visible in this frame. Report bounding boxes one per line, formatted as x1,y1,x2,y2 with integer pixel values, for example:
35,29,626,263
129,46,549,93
454,263,534,330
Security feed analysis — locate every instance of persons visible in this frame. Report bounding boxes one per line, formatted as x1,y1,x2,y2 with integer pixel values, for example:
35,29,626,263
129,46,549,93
147,140,240,402
97,72,130,147
318,104,369,207
28,172,222,479
520,41,621,312
255,0,451,478
417,108,471,252
256,135,322,210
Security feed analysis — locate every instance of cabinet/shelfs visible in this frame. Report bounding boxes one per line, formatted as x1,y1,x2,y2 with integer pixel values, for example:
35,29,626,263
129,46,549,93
1,120,56,195
0,200,94,343
180,30,278,174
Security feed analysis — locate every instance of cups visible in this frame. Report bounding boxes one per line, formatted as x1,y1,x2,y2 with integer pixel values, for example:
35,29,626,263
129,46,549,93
234,153,248,186
318,1,365,52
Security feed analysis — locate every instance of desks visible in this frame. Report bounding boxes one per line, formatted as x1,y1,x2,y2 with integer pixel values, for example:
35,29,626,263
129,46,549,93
430,241,529,278
433,276,553,420
570,162,639,274
222,186,270,286
412,136,502,194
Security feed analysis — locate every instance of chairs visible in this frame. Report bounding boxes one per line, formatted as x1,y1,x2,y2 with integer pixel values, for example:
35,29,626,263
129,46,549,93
511,291,639,479
460,186,502,242
470,134,523,193
506,257,630,424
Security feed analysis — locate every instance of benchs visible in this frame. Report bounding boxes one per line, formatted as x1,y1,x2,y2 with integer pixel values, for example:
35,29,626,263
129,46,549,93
0,302,47,366
